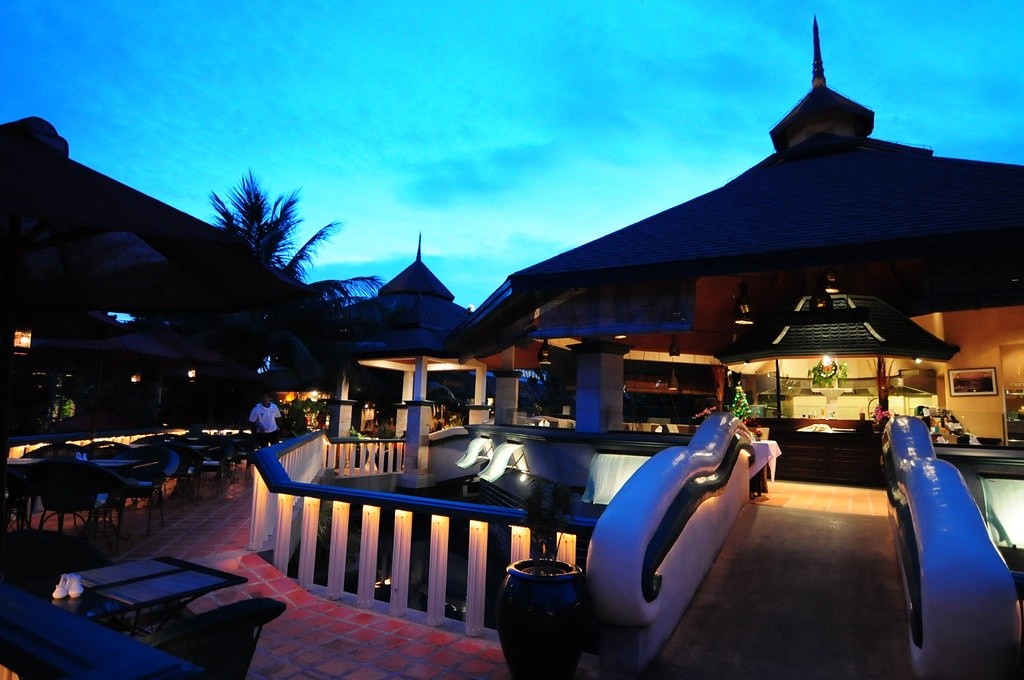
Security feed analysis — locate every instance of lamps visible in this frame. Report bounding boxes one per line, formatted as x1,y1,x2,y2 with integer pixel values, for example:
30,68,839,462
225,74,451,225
669,335,681,356
733,274,754,325
13,325,31,355
668,365,679,391
537,338,553,365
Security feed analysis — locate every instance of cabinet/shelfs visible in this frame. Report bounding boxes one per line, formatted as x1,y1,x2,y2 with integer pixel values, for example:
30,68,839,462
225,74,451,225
1001,385,1024,446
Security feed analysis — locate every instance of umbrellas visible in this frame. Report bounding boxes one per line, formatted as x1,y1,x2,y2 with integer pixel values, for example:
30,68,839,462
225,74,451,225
0,115,325,538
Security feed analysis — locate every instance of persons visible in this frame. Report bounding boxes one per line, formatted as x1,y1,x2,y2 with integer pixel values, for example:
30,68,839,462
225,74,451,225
246,390,281,448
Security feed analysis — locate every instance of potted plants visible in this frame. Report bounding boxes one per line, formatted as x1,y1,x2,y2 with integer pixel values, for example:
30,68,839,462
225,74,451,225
348,425,369,468
374,420,396,473
494,478,590,679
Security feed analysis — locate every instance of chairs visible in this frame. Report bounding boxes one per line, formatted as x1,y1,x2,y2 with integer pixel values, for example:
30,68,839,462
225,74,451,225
0,529,114,600
516,416,770,494
6,432,254,551
139,597,285,680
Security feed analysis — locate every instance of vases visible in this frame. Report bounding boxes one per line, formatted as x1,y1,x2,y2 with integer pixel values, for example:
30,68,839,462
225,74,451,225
756,436,760,441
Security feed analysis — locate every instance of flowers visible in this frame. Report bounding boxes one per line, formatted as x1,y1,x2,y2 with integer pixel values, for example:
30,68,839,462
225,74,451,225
754,431,763,438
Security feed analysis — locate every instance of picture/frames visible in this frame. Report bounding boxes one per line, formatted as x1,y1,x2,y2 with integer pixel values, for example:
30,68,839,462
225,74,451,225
948,366,999,397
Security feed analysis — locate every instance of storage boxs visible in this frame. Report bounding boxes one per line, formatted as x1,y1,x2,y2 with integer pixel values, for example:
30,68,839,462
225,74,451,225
915,406,970,444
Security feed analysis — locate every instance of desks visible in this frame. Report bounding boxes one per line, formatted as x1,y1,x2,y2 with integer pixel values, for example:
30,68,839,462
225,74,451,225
187,438,245,484
6,457,142,539
749,441,782,498
124,444,210,505
57,557,248,638
